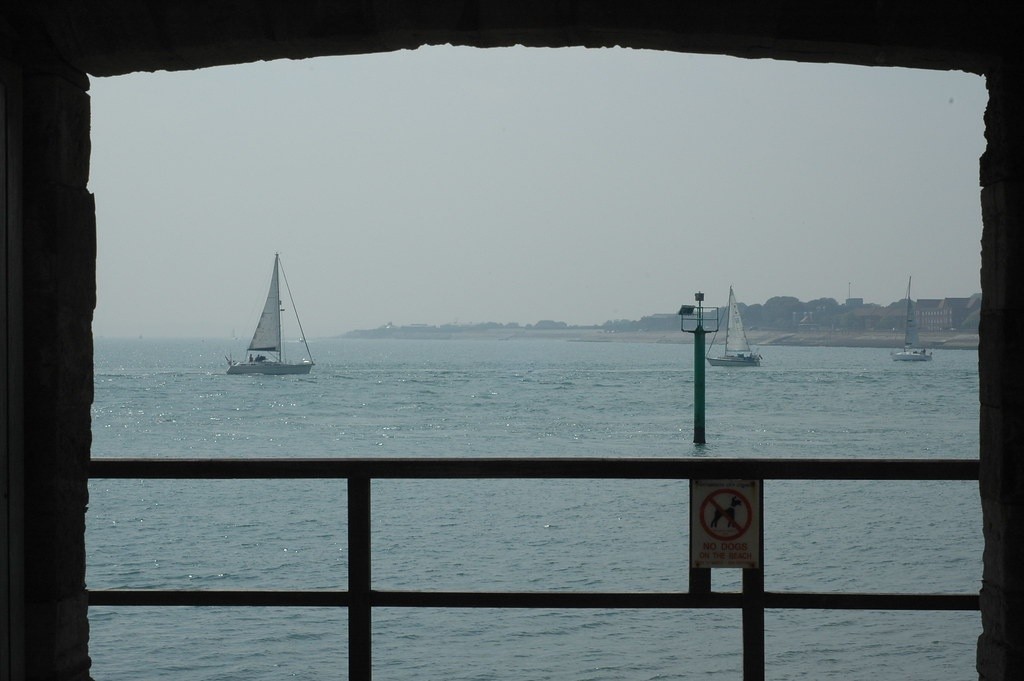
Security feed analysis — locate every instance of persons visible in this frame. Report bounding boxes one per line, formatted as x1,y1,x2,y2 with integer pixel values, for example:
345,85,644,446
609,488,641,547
254,355,263,362
249,354,253,362
923,348,926,353
750,351,752,357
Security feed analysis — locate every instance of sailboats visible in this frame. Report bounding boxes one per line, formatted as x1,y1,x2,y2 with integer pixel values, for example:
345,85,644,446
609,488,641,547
225,252,315,375
890,276,932,361
706,285,762,367
